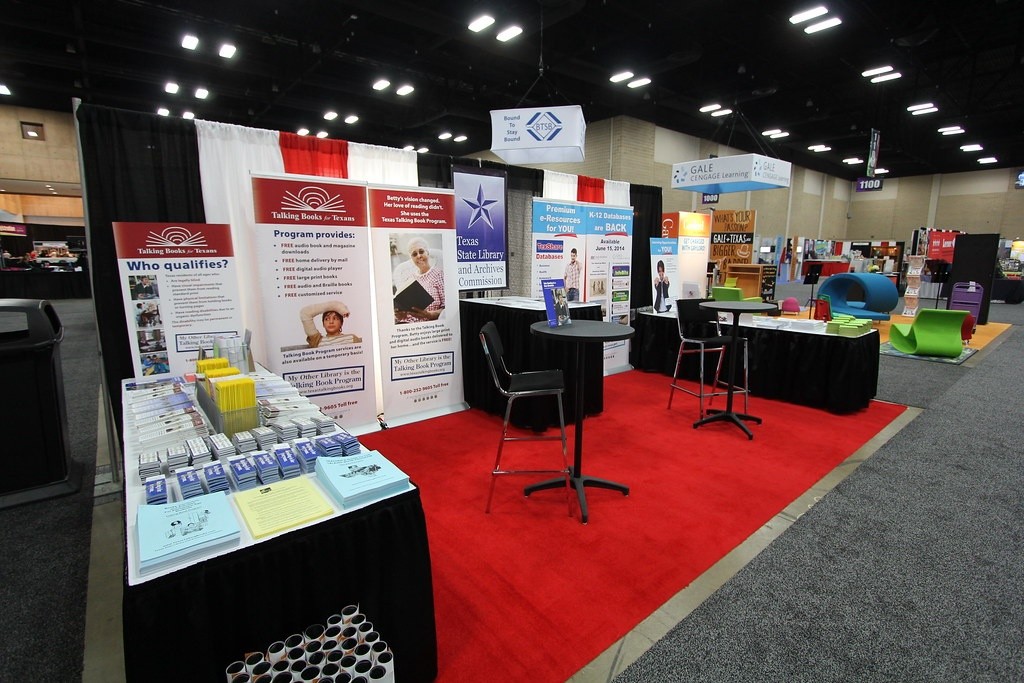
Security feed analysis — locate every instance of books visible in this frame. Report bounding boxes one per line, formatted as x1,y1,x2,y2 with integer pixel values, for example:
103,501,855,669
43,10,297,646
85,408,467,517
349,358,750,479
125,357,319,449
394,280,435,313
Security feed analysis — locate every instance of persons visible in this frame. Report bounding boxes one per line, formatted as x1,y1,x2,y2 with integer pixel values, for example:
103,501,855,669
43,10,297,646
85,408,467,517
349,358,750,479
563,248,582,301
653,260,672,314
131,276,154,300
393,238,446,324
555,294,572,326
136,302,156,327
143,355,159,366
300,299,362,348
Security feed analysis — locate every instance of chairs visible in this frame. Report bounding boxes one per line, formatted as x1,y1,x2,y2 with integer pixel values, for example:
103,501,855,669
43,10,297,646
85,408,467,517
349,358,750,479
889,308,970,358
818,294,851,318
712,286,762,316
814,300,832,322
478,320,574,518
667,299,751,427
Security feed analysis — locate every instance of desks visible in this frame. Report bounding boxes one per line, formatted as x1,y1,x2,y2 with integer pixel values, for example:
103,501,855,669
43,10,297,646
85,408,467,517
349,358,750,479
459,295,604,435
523,320,628,525
120,373,438,683
632,311,880,415
693,300,778,441
919,275,944,300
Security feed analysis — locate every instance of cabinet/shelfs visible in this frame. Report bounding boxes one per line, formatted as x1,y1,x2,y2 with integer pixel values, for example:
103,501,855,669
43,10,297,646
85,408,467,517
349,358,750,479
726,264,777,316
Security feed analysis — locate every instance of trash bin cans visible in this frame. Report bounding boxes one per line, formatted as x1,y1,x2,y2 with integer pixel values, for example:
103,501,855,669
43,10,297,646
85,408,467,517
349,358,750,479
0,297,82,506
